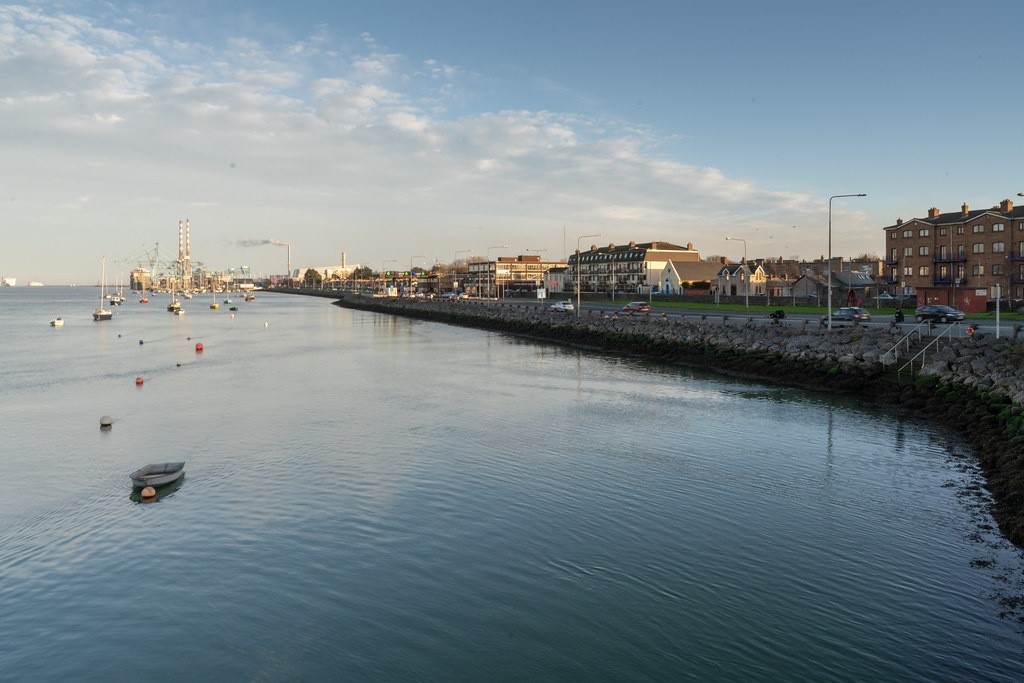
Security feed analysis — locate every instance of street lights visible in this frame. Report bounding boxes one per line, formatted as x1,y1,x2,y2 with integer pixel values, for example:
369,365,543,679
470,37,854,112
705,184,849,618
575,233,599,317
824,192,868,330
726,235,747,313
374,242,550,304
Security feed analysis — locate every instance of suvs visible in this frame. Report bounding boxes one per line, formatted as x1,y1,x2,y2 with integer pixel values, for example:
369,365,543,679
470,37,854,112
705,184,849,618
549,301,574,313
819,306,871,328
622,301,651,315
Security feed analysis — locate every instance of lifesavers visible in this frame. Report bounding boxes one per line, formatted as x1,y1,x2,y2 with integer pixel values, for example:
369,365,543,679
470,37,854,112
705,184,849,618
965,327,974,336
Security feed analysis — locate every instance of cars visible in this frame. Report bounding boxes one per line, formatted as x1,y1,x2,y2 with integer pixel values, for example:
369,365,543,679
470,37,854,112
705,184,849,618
50,317,64,327
128,461,187,489
914,304,965,324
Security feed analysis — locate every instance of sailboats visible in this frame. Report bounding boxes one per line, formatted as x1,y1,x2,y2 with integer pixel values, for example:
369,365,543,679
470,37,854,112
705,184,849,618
92,255,256,319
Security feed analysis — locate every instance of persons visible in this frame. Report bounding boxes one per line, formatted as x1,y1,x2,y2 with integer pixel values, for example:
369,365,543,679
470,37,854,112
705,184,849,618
894,307,905,323
